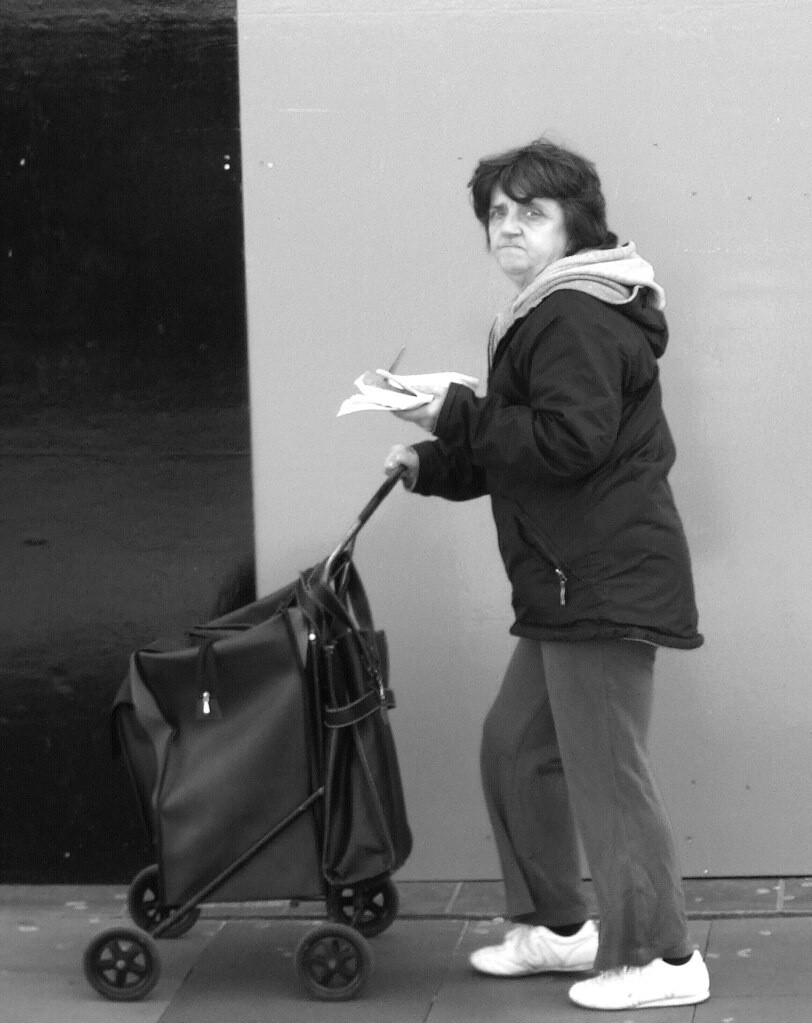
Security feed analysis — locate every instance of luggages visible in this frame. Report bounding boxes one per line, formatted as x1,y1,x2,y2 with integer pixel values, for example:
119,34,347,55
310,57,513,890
86,455,407,1001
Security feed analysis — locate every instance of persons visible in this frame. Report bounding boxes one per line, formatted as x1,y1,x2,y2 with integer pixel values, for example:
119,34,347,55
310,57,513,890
381,141,713,1012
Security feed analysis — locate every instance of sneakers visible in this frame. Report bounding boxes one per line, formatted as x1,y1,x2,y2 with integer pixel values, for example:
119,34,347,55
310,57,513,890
467,918,600,977
568,947,712,1010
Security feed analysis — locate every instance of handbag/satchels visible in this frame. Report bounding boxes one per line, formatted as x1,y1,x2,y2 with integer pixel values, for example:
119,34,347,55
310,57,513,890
300,550,414,886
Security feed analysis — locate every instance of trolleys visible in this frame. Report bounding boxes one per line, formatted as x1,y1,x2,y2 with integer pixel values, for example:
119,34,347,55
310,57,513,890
82,463,408,1000
